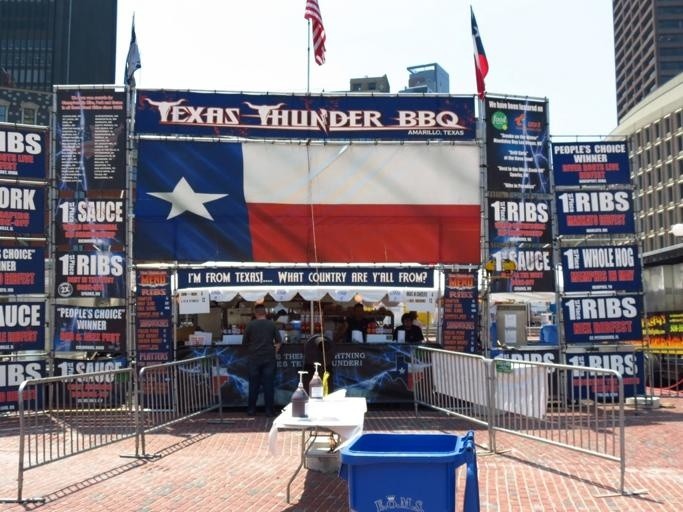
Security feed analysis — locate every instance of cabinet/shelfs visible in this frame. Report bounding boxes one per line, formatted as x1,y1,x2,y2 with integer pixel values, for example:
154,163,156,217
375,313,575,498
220,308,395,343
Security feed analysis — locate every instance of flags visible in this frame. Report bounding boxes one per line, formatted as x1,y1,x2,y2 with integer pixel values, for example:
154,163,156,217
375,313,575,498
122,17,141,91
468,6,490,97
301,1,328,65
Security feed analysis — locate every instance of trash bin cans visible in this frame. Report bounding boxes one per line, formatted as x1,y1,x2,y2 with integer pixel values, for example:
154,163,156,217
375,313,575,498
334,430,480,512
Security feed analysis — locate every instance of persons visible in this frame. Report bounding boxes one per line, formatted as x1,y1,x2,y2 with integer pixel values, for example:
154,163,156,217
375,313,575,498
240,302,282,416
407,309,423,333
345,302,369,342
393,314,424,343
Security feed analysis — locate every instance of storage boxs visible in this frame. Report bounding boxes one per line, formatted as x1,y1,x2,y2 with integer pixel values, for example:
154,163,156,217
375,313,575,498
305,434,341,473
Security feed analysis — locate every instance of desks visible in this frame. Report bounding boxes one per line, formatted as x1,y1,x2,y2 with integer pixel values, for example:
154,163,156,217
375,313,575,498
277,397,366,503
